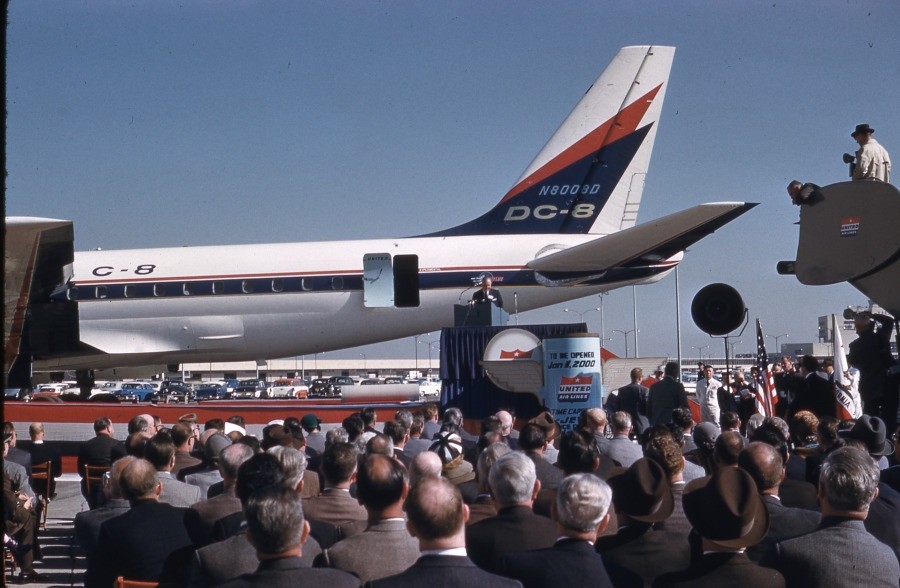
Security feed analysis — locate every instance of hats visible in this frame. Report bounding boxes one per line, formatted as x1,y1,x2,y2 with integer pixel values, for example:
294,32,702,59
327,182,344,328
300,415,319,428
179,414,198,421
527,410,561,442
836,414,896,457
605,456,675,521
851,124,875,137
427,430,463,463
682,464,771,547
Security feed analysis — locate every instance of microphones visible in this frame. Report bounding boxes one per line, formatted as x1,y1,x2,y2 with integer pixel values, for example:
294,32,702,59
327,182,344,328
459,286,473,303
513,292,518,299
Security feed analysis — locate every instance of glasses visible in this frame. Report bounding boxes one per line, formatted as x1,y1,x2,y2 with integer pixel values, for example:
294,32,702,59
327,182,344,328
891,432,899,441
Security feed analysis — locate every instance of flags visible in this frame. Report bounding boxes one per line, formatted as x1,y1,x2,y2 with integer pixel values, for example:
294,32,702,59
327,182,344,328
754,319,778,417
833,314,863,420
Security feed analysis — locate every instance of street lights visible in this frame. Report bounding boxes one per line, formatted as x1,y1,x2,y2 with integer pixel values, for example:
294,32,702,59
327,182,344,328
766,333,790,358
723,341,743,368
411,332,440,383
564,308,640,358
691,344,708,361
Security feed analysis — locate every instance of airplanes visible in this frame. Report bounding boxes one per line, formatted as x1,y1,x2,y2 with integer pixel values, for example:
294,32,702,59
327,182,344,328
6,44,762,389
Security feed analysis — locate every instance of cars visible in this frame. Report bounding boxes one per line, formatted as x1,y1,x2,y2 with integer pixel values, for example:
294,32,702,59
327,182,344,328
7,376,442,399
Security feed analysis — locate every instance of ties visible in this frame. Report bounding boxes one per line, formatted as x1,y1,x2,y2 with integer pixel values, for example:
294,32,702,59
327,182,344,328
707,380,709,388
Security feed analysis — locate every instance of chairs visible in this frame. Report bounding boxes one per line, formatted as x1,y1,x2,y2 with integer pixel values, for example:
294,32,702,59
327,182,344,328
29,459,53,529
85,462,112,509
3,533,17,580
114,574,188,588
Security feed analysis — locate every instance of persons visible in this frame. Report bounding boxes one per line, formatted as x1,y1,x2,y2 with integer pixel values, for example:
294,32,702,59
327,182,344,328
731,372,756,435
849,310,897,441
617,367,650,433
774,354,835,419
852,124,891,184
649,362,690,423
1,407,899,587
472,277,503,308
696,361,705,380
750,367,759,379
717,372,741,414
696,365,723,428
